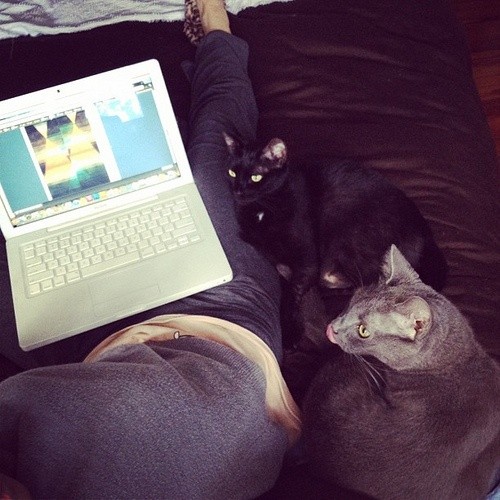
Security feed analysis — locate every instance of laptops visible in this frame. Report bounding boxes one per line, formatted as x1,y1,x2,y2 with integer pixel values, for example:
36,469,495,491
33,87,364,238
0,58,234,351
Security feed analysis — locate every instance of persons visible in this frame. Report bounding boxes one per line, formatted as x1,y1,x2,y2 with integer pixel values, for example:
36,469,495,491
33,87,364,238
0,0,302,500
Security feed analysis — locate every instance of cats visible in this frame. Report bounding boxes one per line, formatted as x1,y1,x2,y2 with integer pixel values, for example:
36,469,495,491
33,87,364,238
219,126,499,500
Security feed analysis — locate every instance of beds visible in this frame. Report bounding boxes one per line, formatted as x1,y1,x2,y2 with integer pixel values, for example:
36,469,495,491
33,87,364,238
0,0,500,500
